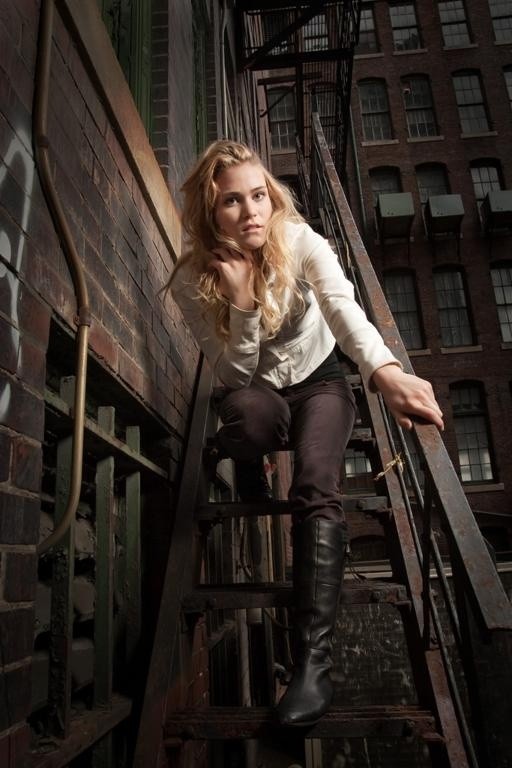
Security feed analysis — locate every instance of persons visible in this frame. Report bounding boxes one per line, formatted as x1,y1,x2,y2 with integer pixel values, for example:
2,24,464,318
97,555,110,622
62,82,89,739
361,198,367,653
168,138,446,728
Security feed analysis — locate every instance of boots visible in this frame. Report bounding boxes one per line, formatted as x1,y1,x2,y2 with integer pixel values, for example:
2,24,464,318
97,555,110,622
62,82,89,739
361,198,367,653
235,453,275,516
273,514,365,727
